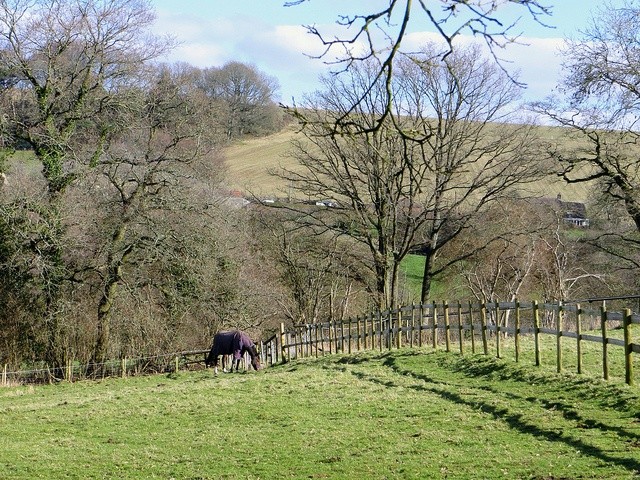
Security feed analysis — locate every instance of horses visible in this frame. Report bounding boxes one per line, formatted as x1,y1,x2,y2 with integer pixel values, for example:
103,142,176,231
204,330,260,374
